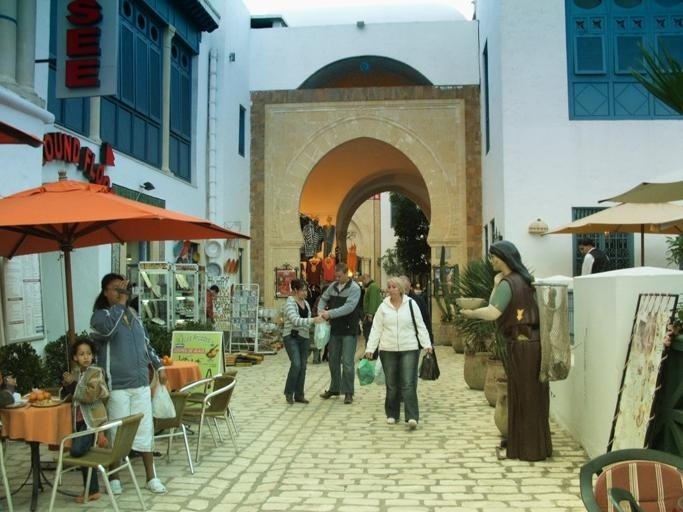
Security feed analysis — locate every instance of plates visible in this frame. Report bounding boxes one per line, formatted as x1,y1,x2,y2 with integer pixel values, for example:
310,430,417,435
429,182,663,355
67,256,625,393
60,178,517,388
30,399,62,407
4,401,27,409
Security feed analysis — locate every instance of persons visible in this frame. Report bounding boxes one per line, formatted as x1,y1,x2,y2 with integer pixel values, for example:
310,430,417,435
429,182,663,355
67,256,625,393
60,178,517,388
59,339,111,503
282,278,324,404
362,277,433,430
316,262,362,404
88,273,168,496
206,285,220,323
578,240,610,276
306,272,434,364
459,240,552,461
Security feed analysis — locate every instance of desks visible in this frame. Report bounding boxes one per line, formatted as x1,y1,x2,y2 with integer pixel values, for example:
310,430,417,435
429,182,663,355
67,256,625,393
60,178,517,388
158,360,202,393
0,397,74,512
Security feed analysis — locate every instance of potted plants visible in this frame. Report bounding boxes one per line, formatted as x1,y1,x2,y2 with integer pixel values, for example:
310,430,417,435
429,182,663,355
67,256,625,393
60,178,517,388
432,244,460,347
493,309,510,438
448,260,483,354
448,255,498,392
466,254,538,407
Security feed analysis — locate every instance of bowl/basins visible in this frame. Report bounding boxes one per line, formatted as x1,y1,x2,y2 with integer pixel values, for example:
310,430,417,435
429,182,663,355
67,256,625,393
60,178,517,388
456,297,483,311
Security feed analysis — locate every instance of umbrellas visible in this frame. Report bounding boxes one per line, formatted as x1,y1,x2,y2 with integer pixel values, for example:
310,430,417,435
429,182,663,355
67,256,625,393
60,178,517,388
540,202,683,267
1,170,251,347
598,180,682,203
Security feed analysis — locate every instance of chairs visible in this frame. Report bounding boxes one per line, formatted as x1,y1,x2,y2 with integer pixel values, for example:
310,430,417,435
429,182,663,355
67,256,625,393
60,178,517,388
176,375,241,463
46,411,149,512
580,447,683,512
145,391,195,480
186,369,240,444
607,487,645,512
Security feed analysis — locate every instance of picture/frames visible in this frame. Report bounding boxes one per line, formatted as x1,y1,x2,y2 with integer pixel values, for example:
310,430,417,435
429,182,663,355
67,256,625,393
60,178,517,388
432,264,460,297
274,267,297,299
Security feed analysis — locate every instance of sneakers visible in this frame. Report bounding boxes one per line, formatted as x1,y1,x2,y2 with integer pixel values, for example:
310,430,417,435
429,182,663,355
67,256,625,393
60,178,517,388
386,418,395,424
109,480,121,495
296,399,308,403
145,478,165,492
344,393,353,404
286,395,294,404
76,493,100,502
409,420,417,430
98,432,108,447
320,391,339,398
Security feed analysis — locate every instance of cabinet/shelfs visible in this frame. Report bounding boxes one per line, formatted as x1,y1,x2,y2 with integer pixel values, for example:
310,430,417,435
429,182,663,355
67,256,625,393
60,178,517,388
137,260,200,335
